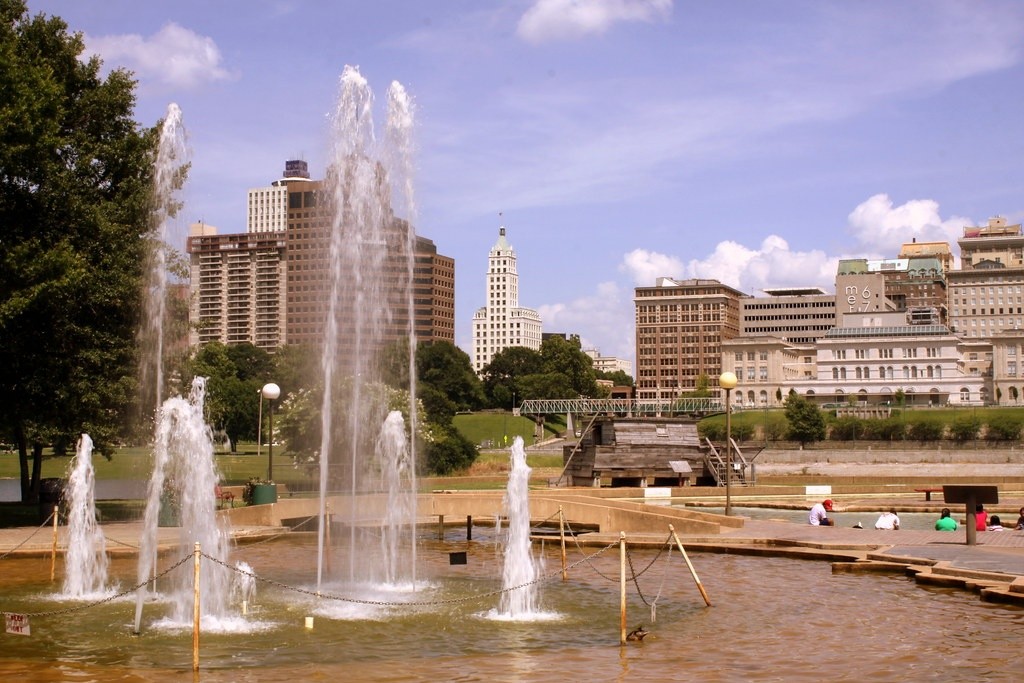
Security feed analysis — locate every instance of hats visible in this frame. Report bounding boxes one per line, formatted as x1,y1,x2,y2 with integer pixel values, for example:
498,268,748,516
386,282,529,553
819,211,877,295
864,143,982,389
824,500,833,510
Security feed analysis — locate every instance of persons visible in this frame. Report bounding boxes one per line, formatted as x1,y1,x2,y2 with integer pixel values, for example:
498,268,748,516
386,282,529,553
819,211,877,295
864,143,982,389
874,508,900,530
809,500,835,526
975,504,988,531
1013,506,1024,530
934,508,957,531
988,515,1003,531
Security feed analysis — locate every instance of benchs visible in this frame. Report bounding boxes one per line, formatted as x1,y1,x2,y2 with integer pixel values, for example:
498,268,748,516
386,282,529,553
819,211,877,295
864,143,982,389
215,483,236,510
915,487,943,501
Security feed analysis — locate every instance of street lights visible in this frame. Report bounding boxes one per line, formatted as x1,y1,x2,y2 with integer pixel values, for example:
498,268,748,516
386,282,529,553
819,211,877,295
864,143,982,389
262,383,280,484
720,371,738,516
